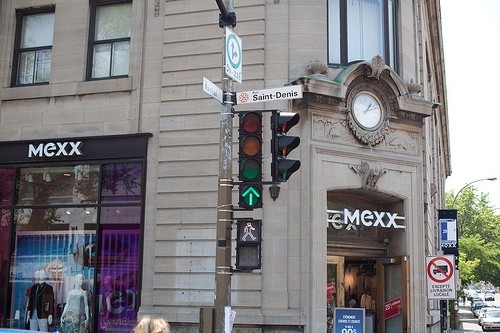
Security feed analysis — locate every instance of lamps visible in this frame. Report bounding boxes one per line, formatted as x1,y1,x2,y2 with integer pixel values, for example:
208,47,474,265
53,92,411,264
25,172,33,182
43,171,51,181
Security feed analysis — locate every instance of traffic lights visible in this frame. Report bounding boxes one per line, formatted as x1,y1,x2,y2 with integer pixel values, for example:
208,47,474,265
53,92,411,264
238,110,263,208
235,219,262,269
270,108,302,182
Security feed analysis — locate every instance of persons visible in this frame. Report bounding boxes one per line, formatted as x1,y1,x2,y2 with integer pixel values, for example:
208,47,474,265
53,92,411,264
26,270,53,332
60,274,90,333
24,271,40,330
103,276,113,328
133,315,173,333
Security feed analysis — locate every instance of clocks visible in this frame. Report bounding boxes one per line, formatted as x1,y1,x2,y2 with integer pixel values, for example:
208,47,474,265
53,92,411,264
352,91,385,131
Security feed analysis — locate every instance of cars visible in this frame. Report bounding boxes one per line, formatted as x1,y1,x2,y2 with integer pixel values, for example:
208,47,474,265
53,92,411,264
467,292,499,332
484,288,496,293
476,289,481,293
485,293,494,301
